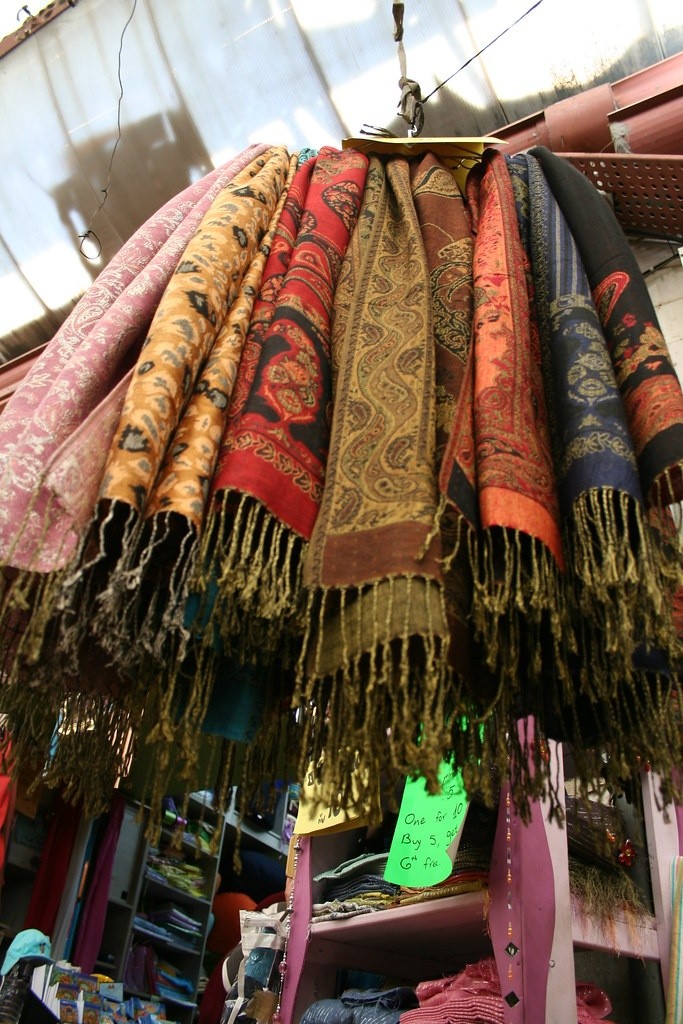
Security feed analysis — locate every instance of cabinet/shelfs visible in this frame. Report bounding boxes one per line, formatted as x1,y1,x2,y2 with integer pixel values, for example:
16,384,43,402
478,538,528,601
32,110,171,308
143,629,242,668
115,793,226,1024
278,718,683,1024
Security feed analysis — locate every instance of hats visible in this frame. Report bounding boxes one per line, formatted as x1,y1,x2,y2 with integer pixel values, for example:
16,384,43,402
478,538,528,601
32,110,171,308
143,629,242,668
0,928,56,976
